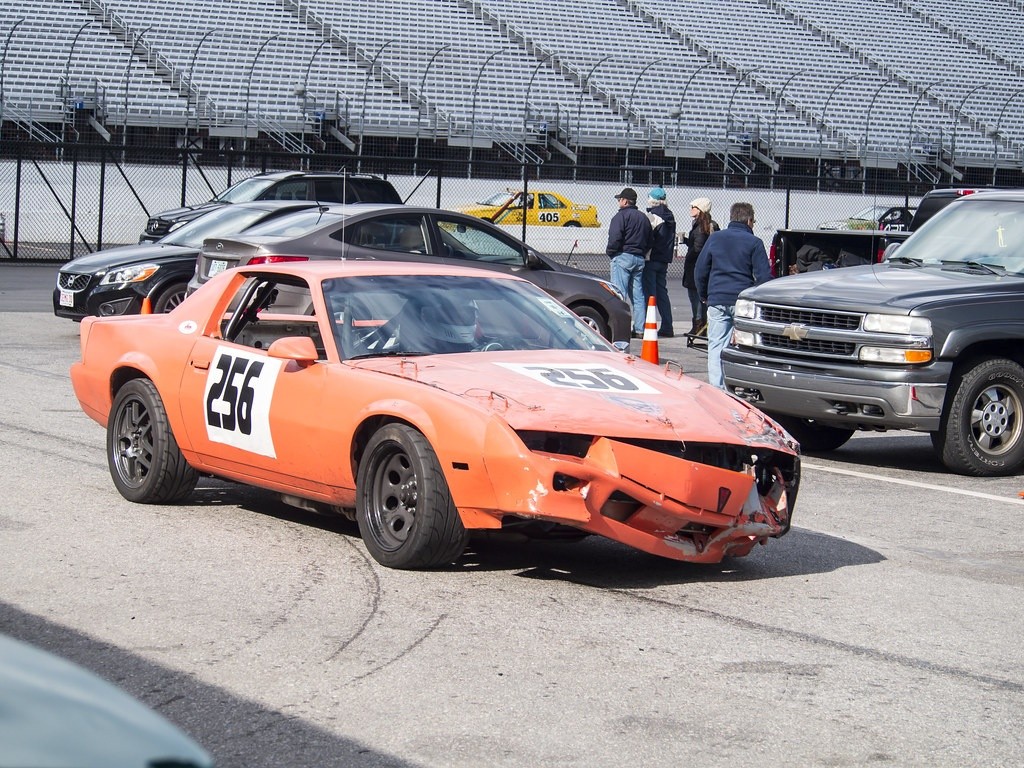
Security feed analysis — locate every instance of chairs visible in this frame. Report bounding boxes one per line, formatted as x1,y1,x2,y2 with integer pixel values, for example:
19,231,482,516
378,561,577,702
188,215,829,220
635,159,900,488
0,0,1024,160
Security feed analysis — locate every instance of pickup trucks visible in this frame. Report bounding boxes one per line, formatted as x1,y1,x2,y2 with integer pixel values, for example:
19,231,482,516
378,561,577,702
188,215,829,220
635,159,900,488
770,188,1003,280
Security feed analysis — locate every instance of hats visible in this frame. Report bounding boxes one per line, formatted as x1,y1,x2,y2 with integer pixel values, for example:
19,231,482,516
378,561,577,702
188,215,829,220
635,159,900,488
649,188,666,200
615,188,636,200
689,198,712,214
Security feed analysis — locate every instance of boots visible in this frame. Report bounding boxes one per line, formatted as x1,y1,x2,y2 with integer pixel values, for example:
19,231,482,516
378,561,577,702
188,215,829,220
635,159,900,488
684,317,707,336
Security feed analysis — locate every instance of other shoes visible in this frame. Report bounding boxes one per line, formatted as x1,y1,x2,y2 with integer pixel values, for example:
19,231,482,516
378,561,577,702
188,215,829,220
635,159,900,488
630,330,644,339
657,329,673,337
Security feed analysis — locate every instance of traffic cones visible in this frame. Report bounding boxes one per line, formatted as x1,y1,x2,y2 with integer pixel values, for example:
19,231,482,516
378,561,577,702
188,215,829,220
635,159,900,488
642,296,658,365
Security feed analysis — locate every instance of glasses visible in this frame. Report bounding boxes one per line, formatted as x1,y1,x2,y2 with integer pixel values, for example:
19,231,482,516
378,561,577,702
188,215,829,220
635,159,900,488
691,206,696,209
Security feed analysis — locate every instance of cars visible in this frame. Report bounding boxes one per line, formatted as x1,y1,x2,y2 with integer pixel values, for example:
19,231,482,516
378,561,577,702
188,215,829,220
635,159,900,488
184,191,632,344
138,171,403,244
53,200,345,323
71,260,801,571
452,188,601,228
816,204,918,232
720,191,1024,477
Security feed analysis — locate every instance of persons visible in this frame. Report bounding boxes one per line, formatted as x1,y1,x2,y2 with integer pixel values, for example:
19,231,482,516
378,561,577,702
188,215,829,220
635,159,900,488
605,188,652,339
400,287,516,354
642,187,674,337
676,198,720,335
693,202,774,386
